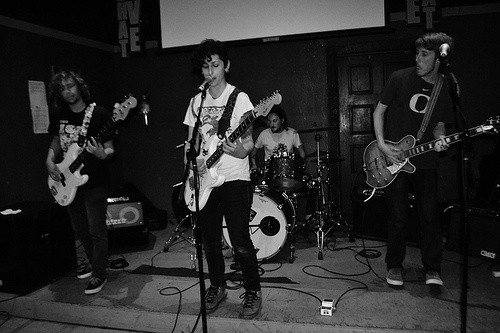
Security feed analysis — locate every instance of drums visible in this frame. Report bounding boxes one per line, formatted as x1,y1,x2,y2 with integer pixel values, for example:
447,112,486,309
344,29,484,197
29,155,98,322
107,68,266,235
266,151,306,190
223,186,297,264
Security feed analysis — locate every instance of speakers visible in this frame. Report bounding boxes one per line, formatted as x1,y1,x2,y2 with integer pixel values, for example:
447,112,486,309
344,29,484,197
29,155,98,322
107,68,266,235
360,189,423,248
105,201,146,230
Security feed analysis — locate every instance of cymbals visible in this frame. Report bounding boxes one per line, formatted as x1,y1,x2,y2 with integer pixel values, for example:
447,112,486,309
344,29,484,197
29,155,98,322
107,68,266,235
305,149,338,157
310,156,347,164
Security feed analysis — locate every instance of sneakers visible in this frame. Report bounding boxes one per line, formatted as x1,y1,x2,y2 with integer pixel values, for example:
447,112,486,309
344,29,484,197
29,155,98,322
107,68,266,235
425,270,444,286
386,267,404,286
84,276,108,295
238,290,262,319
205,283,227,314
76,263,94,280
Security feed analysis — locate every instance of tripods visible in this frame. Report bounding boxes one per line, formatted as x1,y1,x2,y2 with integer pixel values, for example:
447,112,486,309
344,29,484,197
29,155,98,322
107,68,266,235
163,211,196,254
289,130,356,261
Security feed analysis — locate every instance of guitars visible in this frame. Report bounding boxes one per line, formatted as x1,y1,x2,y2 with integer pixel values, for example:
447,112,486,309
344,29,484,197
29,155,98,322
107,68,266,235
47,93,140,207
183,86,284,212
362,117,500,189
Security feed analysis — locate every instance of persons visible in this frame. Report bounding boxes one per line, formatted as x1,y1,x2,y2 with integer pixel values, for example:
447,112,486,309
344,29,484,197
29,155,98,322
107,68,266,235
374,33,453,287
251,106,305,169
182,39,262,320
46,71,116,294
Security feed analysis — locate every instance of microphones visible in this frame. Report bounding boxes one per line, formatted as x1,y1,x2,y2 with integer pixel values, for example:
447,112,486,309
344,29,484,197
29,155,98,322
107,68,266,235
439,42,451,57
198,76,213,91
